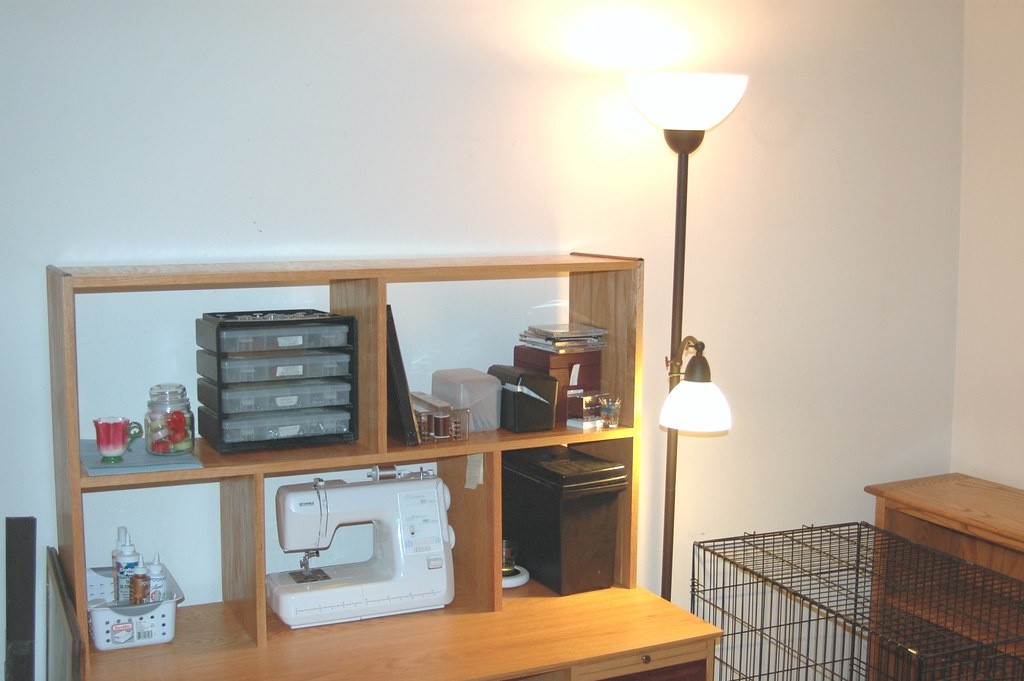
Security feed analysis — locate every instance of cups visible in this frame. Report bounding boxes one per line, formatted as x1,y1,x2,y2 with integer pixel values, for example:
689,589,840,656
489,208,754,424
599,395,622,428
93,417,143,465
449,410,469,441
501,540,519,576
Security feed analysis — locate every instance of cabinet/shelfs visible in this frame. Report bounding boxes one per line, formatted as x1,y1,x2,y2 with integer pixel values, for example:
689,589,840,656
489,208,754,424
863,472,1024,681
45,254,724,681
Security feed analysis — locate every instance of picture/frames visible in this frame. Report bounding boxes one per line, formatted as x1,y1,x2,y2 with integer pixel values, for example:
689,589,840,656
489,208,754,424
45,546,84,681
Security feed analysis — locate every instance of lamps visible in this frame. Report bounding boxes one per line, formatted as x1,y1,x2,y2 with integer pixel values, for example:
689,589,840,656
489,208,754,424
638,66,750,603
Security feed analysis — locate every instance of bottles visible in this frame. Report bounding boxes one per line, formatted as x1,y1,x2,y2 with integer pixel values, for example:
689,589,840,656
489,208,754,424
145,384,195,455
112,527,127,606
117,534,143,607
130,554,150,605
147,553,164,602
